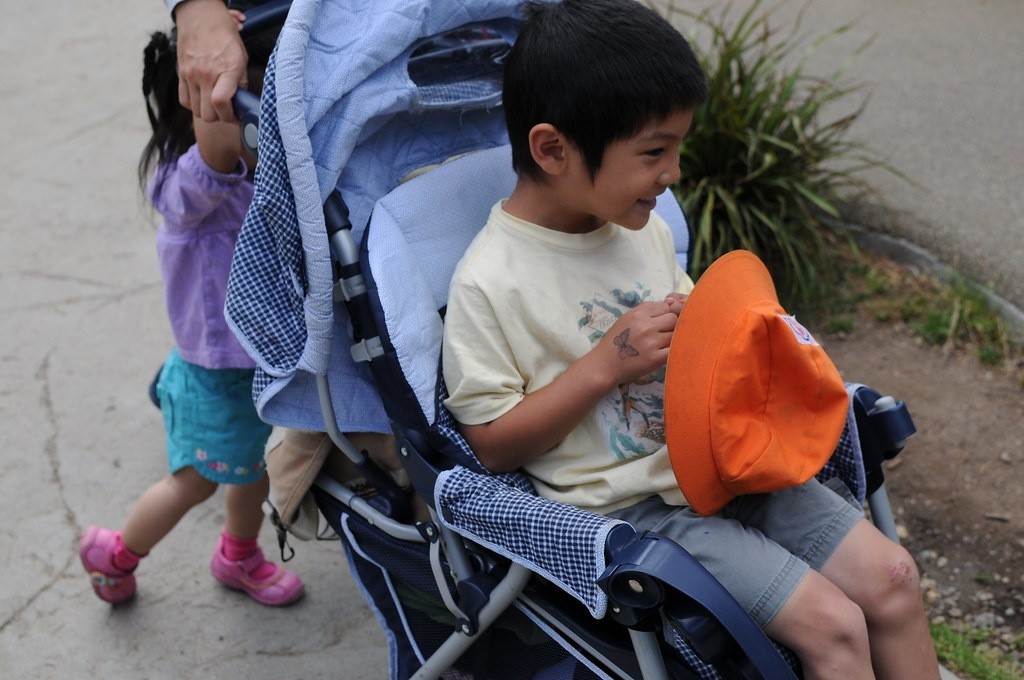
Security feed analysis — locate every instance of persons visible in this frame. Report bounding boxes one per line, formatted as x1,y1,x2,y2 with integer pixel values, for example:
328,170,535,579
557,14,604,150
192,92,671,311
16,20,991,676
163,0,295,123
79,10,308,611
442,0,941,680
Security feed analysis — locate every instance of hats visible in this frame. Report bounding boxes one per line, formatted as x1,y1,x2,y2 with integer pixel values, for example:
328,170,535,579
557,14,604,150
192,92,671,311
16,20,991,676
663,250,849,516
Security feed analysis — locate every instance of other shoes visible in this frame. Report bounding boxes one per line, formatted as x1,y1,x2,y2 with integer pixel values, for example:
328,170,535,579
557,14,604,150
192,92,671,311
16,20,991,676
79,525,137,604
210,536,306,606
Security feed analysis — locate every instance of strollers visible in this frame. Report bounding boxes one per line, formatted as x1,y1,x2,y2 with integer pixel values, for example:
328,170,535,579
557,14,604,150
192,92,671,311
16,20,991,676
187,1,919,679
